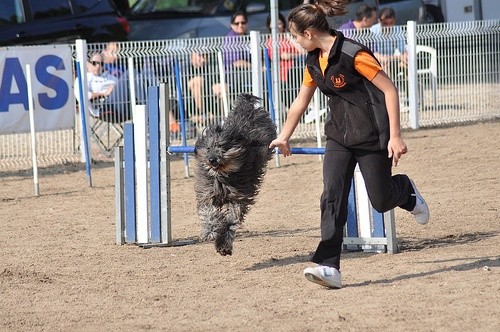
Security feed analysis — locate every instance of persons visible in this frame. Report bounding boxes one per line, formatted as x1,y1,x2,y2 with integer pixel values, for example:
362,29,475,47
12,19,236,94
222,4,410,125
74,42,230,137
269,0,430,290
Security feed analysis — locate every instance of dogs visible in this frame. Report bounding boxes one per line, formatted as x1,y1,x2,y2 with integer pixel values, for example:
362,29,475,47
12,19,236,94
193,94,277,256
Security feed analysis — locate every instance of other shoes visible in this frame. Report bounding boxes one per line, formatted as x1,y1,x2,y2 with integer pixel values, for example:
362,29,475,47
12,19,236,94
170,129,188,139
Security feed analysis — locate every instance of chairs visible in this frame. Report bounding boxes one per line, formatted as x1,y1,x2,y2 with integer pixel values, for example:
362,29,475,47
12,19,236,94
74,44,437,118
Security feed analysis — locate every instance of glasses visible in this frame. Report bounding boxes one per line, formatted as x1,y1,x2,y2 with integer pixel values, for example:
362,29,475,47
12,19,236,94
234,22,247,25
89,60,103,66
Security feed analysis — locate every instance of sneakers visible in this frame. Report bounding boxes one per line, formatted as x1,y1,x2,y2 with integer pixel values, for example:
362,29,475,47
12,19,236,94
304,265,342,288
409,178,429,225
